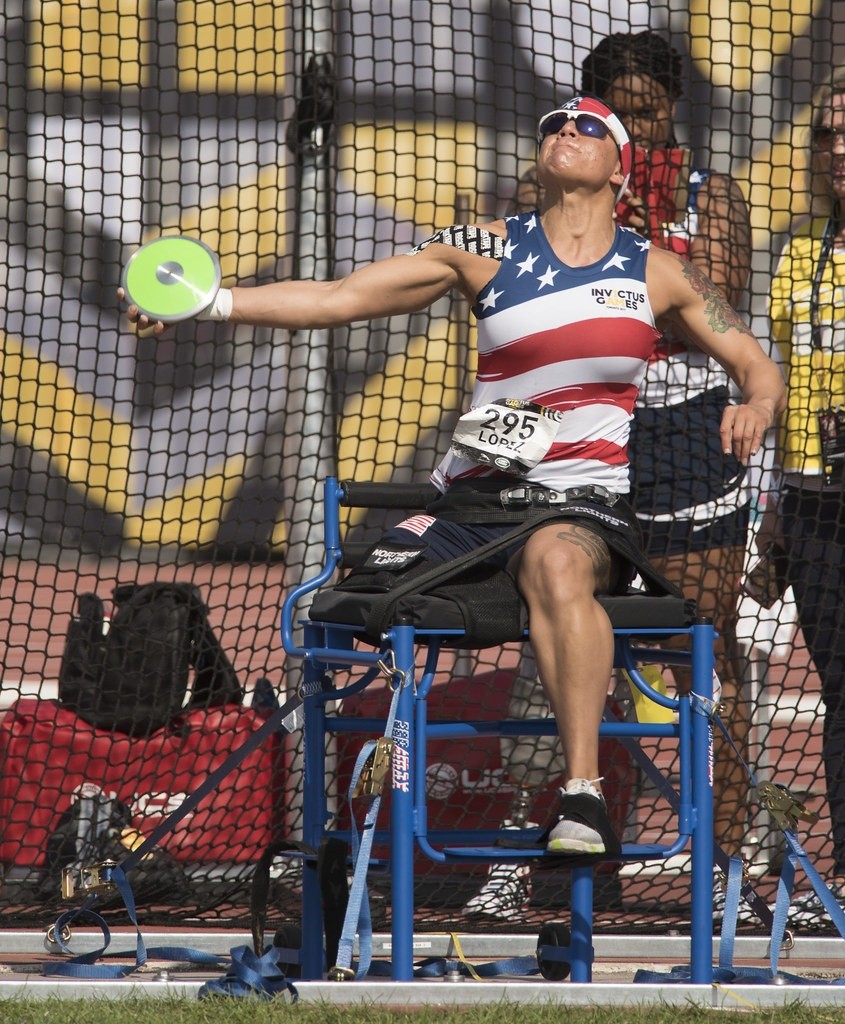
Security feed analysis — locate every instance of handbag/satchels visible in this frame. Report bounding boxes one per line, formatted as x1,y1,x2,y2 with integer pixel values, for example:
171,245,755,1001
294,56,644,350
57,571,243,740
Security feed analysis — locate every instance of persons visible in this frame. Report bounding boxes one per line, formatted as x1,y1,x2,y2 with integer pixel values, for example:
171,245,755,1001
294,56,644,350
459,32,845,926
117,97,790,854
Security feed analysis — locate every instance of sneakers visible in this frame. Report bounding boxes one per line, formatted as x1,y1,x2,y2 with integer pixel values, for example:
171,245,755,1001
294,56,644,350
547,777,611,856
462,861,540,921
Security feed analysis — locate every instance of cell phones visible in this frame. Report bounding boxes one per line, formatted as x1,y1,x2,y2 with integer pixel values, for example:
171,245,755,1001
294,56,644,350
740,543,790,610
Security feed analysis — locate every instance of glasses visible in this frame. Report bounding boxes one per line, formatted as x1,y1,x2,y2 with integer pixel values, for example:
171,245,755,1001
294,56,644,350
538,112,611,139
811,126,845,150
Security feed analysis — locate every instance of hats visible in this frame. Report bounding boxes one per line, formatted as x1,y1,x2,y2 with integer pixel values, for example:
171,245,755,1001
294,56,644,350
580,30,685,98
537,96,633,208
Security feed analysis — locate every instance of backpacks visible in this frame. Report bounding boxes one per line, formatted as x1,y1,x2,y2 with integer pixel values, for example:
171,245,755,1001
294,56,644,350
34,792,134,902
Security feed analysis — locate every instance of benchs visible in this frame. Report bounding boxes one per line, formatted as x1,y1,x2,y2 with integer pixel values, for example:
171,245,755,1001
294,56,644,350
280,475,719,986
